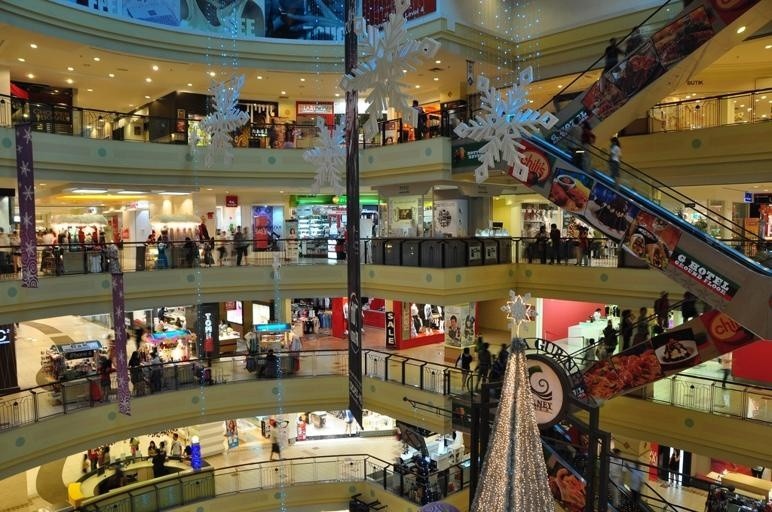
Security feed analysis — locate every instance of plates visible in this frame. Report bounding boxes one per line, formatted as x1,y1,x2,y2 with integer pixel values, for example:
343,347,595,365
584,200,627,240
654,340,698,365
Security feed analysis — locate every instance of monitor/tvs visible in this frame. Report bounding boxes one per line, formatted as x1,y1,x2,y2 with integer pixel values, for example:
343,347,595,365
97,465,105,476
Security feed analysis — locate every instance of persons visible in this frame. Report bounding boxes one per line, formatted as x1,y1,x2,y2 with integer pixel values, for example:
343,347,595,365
601,36,627,78
95,116,106,141
0,223,255,274
625,459,646,512
579,287,711,371
521,220,594,269
666,447,681,488
384,137,394,144
290,228,296,239
606,448,624,509
446,314,461,342
403,301,446,334
628,26,645,54
410,98,426,141
448,337,511,391
565,114,623,193
718,352,734,390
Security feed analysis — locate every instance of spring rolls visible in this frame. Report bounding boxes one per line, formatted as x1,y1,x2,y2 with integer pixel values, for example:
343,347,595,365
630,234,663,268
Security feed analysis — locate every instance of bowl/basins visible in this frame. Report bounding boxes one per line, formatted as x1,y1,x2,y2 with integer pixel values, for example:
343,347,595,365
557,175,576,191
